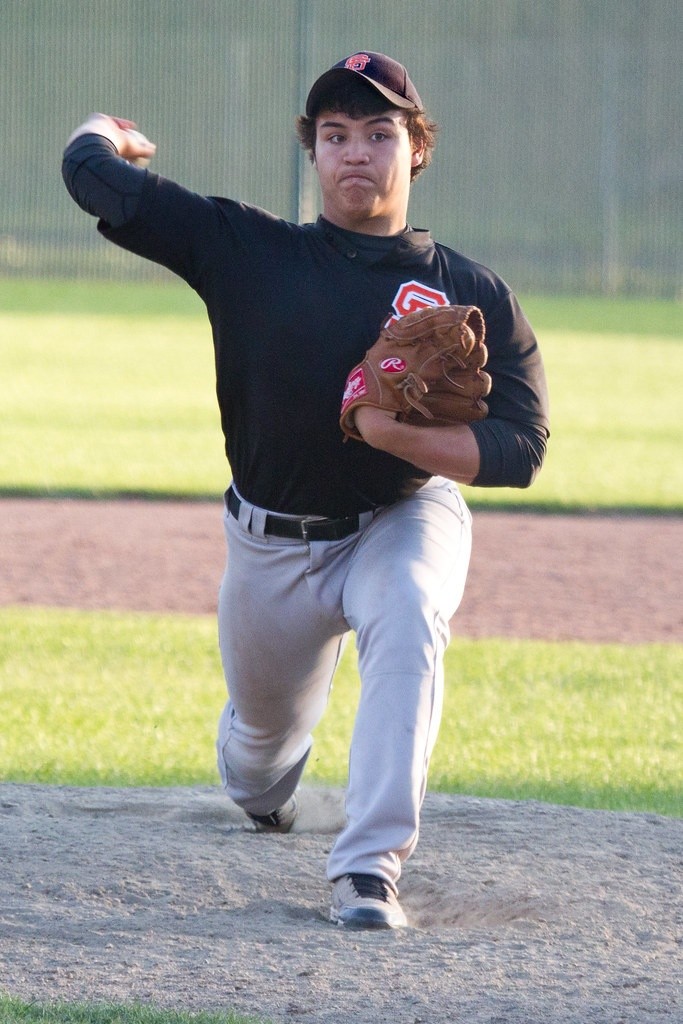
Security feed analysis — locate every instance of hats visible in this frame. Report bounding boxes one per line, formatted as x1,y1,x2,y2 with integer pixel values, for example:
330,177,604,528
305,50,424,117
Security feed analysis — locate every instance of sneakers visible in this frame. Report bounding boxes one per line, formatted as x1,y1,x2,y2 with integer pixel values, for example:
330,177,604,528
330,874,408,930
245,785,299,835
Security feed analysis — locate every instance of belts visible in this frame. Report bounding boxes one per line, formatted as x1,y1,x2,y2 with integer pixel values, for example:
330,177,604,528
227,486,359,541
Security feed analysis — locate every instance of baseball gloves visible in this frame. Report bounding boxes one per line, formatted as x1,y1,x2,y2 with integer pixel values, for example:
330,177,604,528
339,304,493,443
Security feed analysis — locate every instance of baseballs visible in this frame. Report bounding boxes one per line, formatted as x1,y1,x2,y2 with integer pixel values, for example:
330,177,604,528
124,129,151,169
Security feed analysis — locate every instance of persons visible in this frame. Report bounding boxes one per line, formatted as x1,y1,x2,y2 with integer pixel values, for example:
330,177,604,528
62,53,549,926
